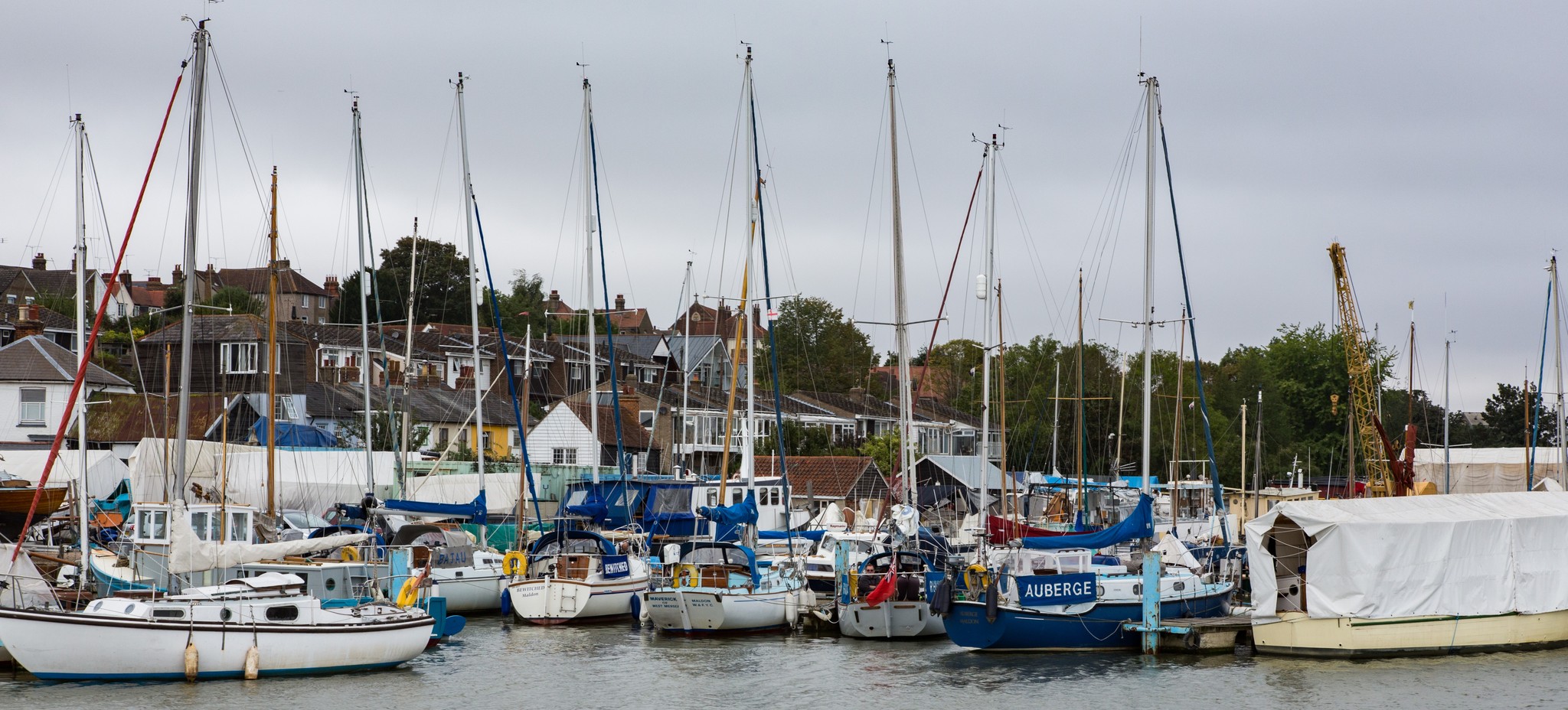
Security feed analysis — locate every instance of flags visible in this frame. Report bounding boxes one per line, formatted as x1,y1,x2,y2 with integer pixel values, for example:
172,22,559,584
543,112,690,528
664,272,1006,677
866,555,897,607
517,311,529,317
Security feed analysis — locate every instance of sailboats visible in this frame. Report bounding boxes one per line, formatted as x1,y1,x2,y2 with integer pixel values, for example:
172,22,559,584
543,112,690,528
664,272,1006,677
0,12,1568,684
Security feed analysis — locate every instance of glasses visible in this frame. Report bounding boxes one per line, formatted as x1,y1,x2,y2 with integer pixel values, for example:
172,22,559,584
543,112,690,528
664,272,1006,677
866,569,874,572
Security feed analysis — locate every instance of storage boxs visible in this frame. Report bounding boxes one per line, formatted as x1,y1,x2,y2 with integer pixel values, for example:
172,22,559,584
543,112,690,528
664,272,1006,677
1273,524,1308,576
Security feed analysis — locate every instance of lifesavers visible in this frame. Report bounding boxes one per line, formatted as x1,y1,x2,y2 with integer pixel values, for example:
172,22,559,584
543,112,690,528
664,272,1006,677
341,546,362,561
671,562,699,588
850,570,858,597
1183,631,1201,651
502,551,527,575
395,577,418,608
964,563,991,592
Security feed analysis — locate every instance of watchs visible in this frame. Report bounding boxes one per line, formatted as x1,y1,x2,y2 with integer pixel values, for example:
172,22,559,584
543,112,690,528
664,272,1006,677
874,586,876,588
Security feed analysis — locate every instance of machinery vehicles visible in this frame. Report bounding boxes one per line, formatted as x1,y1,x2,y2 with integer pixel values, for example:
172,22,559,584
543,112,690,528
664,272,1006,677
1326,240,1438,498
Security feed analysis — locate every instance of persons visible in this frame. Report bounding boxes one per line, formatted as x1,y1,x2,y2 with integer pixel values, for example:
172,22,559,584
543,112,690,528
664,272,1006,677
858,564,880,597
68,534,81,550
1195,510,1198,518
615,541,628,555
1060,498,1072,524
